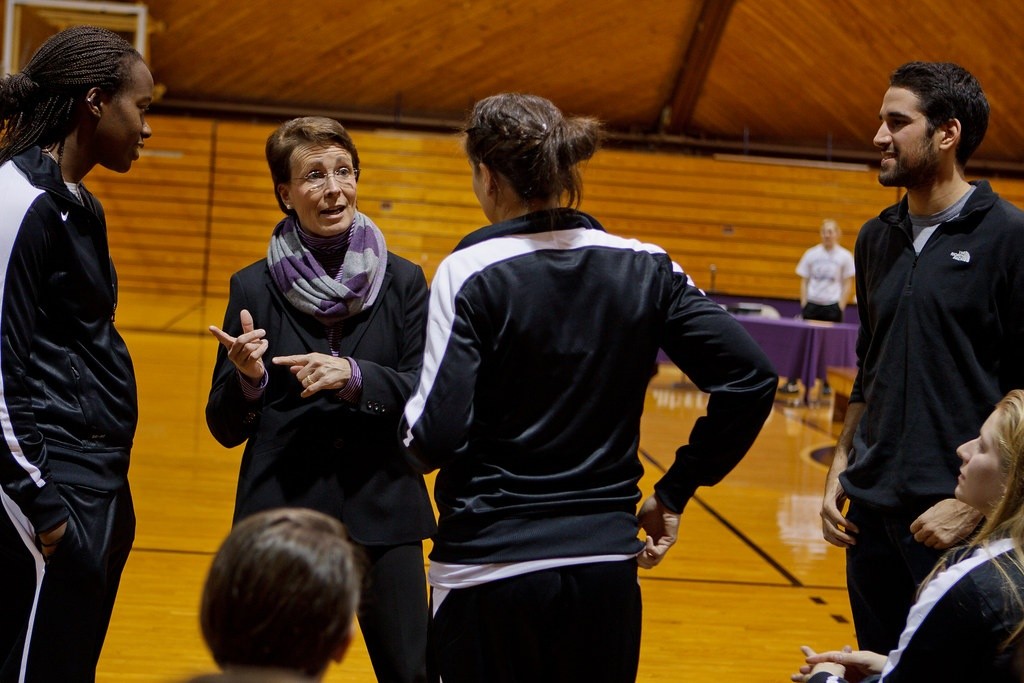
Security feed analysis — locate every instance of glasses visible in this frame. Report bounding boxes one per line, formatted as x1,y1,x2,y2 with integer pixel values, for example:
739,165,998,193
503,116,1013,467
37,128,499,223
290,166,361,186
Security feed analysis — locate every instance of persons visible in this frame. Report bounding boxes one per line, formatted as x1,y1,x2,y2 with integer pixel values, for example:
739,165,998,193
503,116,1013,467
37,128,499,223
171,508,362,683
792,61,1024,683
396,92,780,683
0,23,157,683
206,113,446,683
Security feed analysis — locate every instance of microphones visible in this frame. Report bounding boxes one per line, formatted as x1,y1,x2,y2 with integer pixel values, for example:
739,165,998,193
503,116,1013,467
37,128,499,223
710,263,717,290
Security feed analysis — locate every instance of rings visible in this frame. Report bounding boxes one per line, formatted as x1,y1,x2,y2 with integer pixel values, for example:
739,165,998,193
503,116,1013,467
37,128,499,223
306,374,314,385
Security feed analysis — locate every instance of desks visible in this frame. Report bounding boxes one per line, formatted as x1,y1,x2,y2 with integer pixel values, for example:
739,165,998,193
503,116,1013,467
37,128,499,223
678,311,863,405
653,289,867,367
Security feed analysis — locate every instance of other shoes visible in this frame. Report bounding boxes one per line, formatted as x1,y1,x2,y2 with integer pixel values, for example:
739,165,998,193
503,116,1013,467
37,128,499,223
779,383,798,395
822,386,830,395
